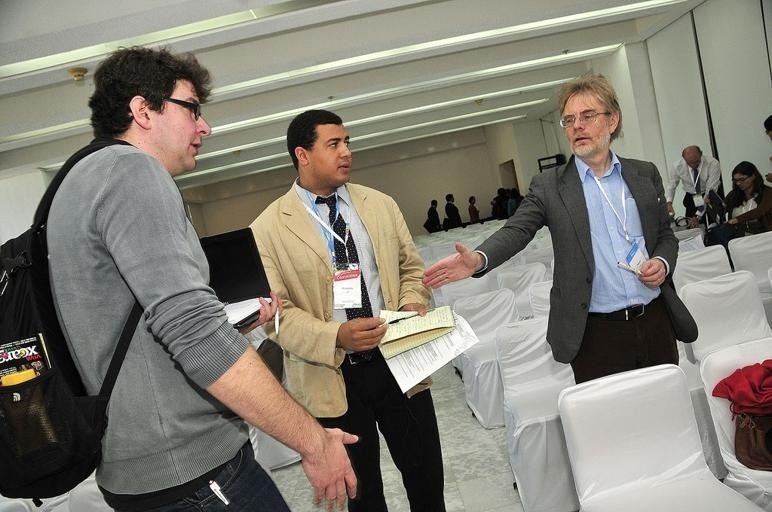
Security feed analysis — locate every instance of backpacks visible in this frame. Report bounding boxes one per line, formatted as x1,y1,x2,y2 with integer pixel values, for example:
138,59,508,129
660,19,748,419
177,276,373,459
1,139,144,509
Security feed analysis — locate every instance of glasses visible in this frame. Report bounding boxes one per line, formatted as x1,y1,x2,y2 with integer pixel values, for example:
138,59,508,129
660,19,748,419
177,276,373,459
732,175,752,182
559,113,611,129
160,97,201,121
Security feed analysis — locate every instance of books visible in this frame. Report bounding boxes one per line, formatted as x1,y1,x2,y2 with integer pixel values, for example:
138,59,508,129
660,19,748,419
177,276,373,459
379,304,456,361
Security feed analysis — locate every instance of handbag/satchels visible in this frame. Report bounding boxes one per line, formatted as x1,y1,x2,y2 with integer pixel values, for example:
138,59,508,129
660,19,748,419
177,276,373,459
734,414,772,471
704,222,746,268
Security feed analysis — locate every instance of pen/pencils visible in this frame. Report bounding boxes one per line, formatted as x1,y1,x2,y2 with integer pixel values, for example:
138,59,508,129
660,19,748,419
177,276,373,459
275,307,279,336
209,479,230,506
618,262,643,275
388,308,435,324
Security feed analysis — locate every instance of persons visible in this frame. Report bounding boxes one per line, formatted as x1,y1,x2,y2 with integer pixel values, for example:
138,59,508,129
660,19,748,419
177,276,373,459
423,187,525,234
47,43,360,512
246,110,447,510
665,113,772,269
421,73,698,384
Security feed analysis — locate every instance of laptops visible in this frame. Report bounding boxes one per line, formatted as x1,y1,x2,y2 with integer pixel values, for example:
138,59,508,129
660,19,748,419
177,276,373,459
200,228,274,329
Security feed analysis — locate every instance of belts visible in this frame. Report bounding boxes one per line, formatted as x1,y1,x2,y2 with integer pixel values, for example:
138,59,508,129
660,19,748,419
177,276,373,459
340,350,384,366
587,295,663,322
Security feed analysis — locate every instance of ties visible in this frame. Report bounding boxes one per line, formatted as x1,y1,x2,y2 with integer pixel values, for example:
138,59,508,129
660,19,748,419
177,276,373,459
315,196,378,363
693,168,701,195
